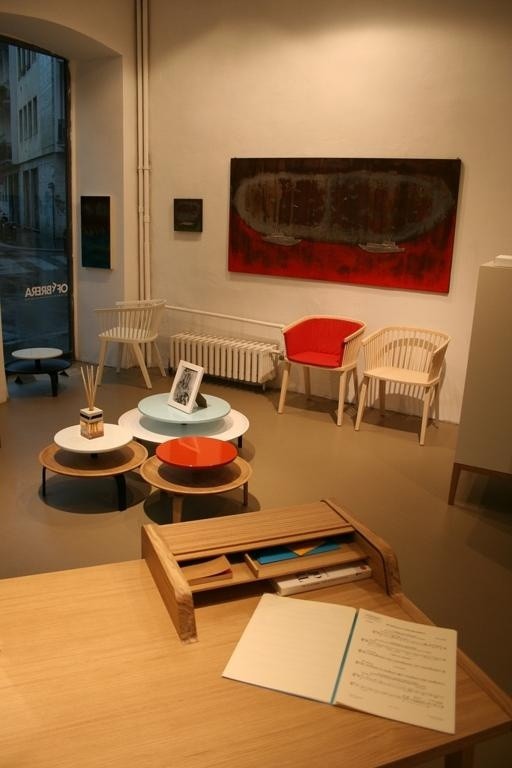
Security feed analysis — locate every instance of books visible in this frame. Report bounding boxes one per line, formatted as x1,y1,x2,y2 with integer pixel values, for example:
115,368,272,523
179,555,234,587
267,560,372,597
223,592,458,736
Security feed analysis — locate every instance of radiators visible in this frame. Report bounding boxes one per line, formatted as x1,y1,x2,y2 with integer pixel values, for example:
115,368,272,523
168,331,283,392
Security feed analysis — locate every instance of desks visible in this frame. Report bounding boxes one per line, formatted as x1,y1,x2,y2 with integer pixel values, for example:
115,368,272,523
0,496,512,768
37,391,252,524
3,347,72,398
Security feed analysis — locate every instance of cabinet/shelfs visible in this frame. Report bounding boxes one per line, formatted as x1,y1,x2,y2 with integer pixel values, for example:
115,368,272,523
447,257,511,503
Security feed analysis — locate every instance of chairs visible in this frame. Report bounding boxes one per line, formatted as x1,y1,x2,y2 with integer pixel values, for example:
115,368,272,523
277,316,368,427
93,299,166,390
354,325,452,447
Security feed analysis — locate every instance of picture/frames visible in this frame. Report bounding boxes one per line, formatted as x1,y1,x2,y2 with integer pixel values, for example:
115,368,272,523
166,359,204,415
